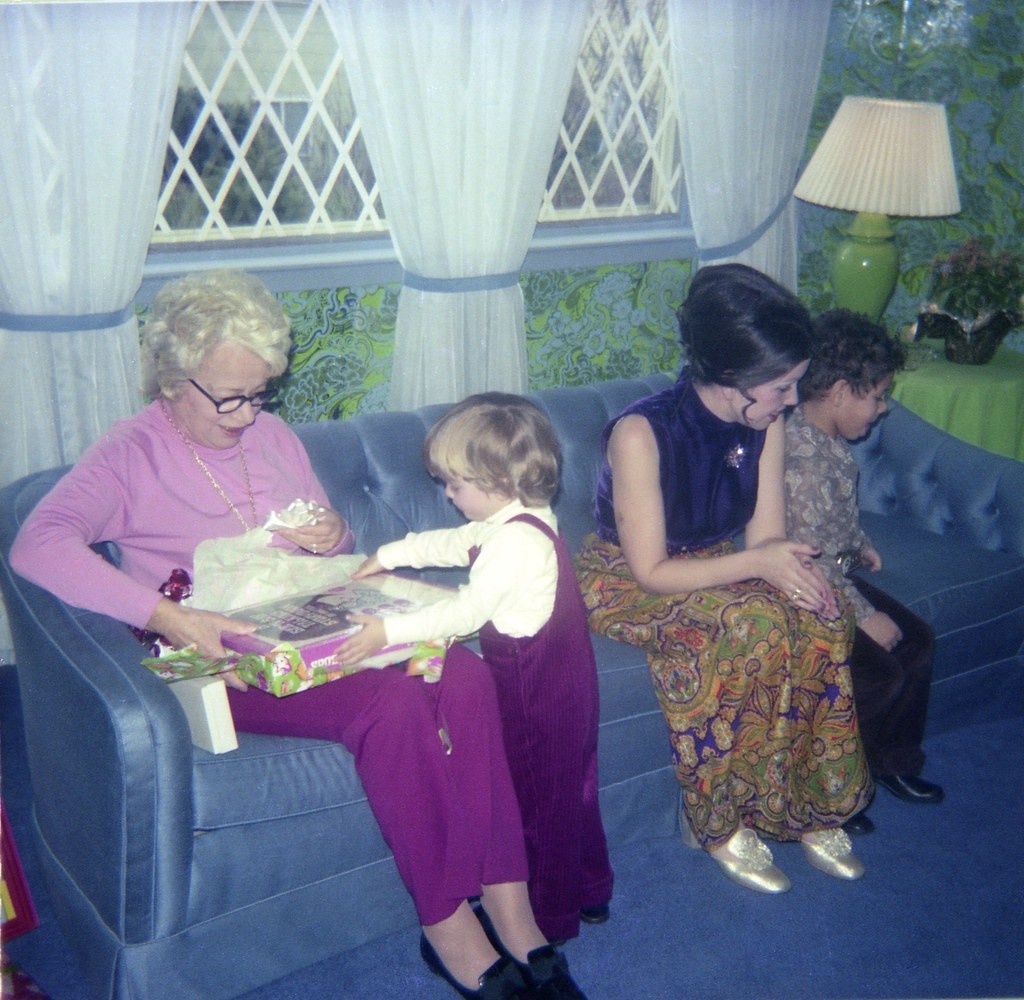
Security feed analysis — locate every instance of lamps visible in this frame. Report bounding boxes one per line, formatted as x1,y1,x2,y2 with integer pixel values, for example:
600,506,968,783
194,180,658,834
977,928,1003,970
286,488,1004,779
792,94,961,321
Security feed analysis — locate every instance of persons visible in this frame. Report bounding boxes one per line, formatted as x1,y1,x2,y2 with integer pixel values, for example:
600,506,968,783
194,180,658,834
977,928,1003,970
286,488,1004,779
9,267,589,1000
334,392,614,947
573,264,874,892
783,306,946,834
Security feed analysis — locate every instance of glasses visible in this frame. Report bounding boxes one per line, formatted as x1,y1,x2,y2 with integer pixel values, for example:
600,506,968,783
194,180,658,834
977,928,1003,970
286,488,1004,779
184,377,280,415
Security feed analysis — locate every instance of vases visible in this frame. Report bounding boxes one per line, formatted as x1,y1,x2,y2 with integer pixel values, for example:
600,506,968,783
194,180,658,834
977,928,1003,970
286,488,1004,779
913,310,1018,365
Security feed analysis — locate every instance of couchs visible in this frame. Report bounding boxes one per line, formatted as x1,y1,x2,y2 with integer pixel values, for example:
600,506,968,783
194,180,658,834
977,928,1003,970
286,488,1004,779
1,372,1022,999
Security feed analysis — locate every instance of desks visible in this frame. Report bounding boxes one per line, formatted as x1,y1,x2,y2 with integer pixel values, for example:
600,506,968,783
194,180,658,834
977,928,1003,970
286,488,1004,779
885,332,1024,466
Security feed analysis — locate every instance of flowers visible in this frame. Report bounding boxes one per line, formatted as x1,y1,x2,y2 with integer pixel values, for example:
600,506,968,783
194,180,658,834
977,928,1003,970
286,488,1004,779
920,238,1023,331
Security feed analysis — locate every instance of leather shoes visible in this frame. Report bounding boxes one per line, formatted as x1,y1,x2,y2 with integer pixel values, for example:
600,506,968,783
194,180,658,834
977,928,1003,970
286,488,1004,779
418,902,587,1000
842,813,875,835
877,771,946,805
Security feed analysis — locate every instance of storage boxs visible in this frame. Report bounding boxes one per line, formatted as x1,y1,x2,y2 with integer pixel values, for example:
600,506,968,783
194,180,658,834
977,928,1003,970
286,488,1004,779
218,572,480,697
169,676,239,755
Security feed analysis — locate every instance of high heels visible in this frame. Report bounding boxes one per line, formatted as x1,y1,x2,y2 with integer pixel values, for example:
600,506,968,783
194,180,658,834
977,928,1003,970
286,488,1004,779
798,827,867,881
679,806,794,894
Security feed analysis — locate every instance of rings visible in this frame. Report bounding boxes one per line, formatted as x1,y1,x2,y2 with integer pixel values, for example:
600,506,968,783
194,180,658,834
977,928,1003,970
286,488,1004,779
313,543,317,553
793,588,801,602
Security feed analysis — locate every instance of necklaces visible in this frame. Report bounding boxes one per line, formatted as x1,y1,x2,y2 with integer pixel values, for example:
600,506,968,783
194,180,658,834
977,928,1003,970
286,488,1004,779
161,395,257,532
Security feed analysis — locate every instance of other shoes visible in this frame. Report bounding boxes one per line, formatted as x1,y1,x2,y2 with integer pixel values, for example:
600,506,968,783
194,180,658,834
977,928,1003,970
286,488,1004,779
580,904,610,925
